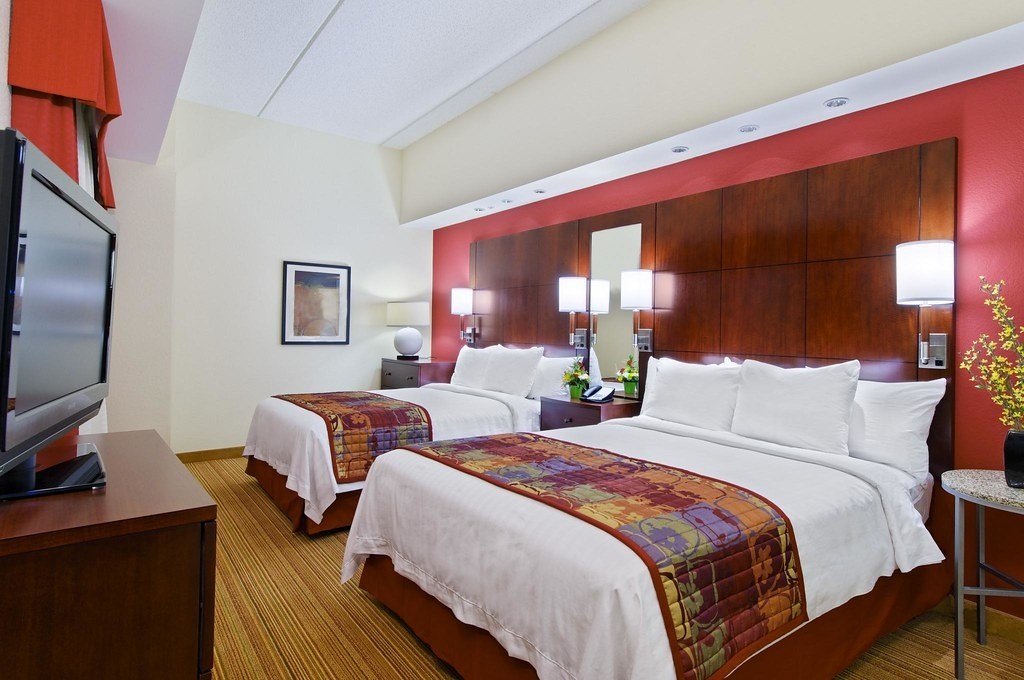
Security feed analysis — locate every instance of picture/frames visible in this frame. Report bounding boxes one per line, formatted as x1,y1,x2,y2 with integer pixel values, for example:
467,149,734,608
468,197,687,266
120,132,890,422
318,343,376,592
282,260,351,345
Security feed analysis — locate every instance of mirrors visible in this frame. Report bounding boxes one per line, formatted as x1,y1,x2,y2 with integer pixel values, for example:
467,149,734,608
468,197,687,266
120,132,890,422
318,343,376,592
591,222,642,398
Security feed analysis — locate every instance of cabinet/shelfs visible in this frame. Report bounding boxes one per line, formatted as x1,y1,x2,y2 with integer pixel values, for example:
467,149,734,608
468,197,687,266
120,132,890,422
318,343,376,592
0,429,217,680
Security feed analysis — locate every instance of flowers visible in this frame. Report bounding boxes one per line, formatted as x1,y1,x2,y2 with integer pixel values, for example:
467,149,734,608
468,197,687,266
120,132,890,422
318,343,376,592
562,352,590,389
616,355,640,383
958,275,1024,432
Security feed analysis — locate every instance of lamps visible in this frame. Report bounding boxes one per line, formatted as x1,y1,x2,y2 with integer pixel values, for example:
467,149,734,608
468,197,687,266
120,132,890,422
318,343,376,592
619,269,652,353
559,277,586,350
591,279,610,345
895,239,954,368
386,287,476,360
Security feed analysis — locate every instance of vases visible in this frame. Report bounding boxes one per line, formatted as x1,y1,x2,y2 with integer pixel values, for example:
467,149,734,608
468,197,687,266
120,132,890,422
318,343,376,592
567,385,583,398
624,381,636,394
1004,430,1024,488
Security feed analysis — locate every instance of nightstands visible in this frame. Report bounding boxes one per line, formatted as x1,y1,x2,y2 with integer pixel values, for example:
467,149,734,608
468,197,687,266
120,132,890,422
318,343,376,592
540,395,640,431
381,358,456,389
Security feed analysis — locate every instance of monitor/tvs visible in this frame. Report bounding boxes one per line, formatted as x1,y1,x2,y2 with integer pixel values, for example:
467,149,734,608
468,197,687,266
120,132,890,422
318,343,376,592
0,127,119,501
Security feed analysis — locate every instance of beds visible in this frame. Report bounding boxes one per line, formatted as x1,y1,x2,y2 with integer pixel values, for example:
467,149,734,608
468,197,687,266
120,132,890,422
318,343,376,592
243,383,540,533
340,417,956,680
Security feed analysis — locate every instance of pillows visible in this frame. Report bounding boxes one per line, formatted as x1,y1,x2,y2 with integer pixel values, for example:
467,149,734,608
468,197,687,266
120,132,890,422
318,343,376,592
639,355,947,481
451,344,586,399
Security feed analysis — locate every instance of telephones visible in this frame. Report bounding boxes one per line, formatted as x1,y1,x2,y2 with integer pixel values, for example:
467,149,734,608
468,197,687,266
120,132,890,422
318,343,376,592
579,386,615,403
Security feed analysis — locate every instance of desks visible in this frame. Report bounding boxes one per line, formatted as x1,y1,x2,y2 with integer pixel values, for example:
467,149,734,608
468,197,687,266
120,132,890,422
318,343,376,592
941,470,1024,680
